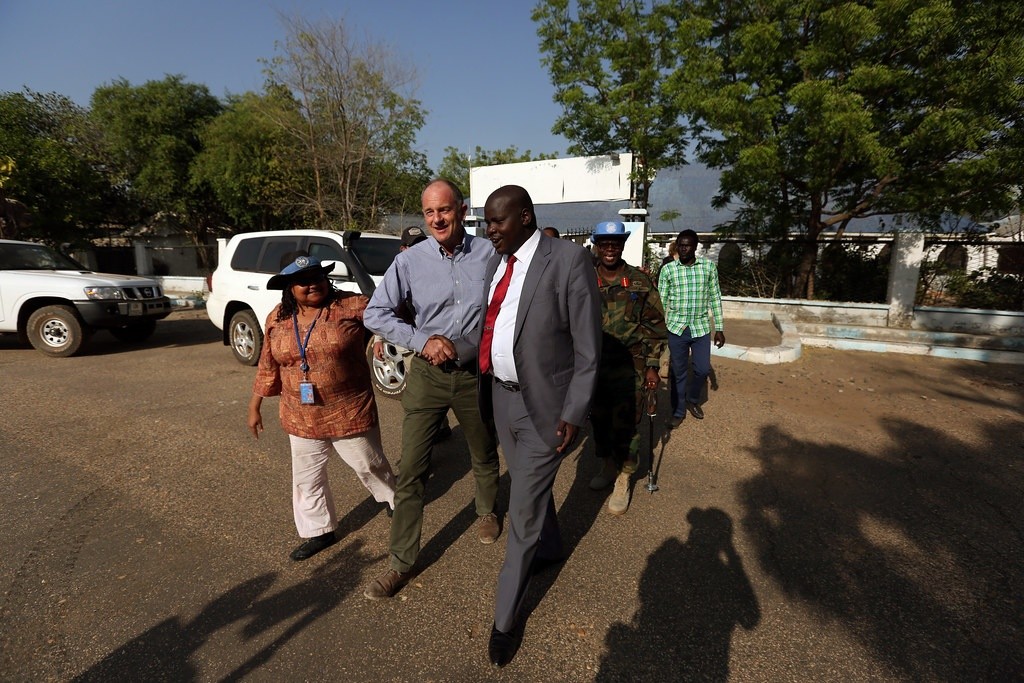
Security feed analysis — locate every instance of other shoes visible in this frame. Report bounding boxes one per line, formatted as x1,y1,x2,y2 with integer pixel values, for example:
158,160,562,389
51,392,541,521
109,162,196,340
686,401,704,419
590,464,617,489
669,416,683,429
387,503,393,518
364,569,409,601
608,477,631,514
290,532,336,560
479,513,500,545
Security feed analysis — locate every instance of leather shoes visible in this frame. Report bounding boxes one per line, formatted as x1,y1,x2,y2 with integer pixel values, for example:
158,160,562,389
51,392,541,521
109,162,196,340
539,524,563,568
489,621,515,667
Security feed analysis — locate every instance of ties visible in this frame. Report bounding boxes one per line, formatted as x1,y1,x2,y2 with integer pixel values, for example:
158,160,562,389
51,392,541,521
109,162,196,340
480,255,516,374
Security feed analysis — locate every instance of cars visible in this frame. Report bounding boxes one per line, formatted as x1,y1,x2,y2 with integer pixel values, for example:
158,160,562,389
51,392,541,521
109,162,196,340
203,228,404,367
0,238,173,358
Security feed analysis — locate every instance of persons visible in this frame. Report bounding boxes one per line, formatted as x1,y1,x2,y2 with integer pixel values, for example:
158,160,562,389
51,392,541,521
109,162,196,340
543,227,560,238
589,221,667,515
248,251,396,560
660,241,680,378
363,181,497,601
657,229,725,429
426,185,603,665
374,227,452,446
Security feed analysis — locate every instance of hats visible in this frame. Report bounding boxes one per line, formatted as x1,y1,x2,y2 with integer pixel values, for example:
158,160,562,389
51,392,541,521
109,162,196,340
267,256,335,290
591,222,631,244
402,226,427,245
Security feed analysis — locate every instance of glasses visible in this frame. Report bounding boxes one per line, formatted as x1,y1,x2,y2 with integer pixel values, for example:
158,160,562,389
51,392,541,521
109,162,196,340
596,242,624,251
